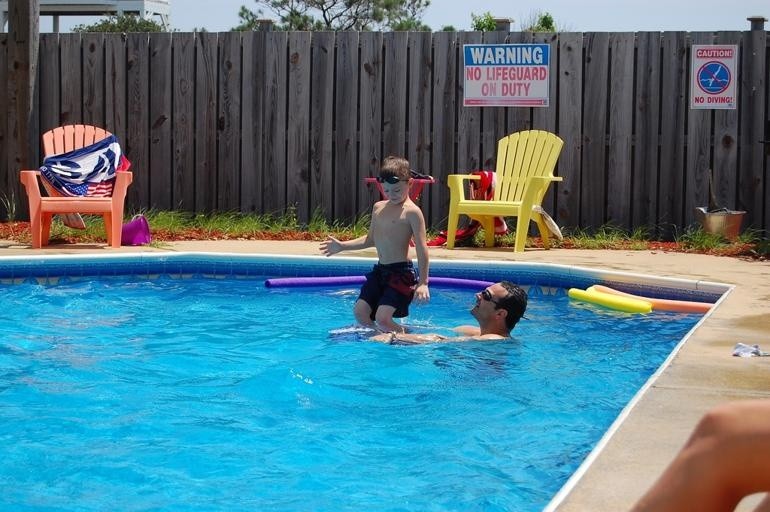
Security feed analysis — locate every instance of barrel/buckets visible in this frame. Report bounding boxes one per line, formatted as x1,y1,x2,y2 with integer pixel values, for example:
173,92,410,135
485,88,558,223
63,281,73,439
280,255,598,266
121,214,151,245
696,206,747,244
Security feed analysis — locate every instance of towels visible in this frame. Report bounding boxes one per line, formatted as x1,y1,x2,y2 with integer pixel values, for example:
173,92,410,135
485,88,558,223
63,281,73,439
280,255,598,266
426,168,508,247
38,135,132,230
733,342,770,358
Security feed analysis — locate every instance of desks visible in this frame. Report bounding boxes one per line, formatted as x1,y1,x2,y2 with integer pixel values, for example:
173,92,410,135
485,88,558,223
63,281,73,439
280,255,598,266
364,176,437,247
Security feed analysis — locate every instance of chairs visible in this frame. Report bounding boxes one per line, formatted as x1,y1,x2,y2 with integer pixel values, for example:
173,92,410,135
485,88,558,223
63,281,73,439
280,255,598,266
19,123,133,248
448,130,564,250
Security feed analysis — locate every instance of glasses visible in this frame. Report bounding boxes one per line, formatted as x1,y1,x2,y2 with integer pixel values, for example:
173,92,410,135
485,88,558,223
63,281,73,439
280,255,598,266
376,175,409,184
482,290,503,309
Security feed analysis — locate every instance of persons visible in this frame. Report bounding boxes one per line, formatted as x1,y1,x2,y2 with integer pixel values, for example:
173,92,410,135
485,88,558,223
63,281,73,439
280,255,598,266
364,279,529,342
318,156,431,333
